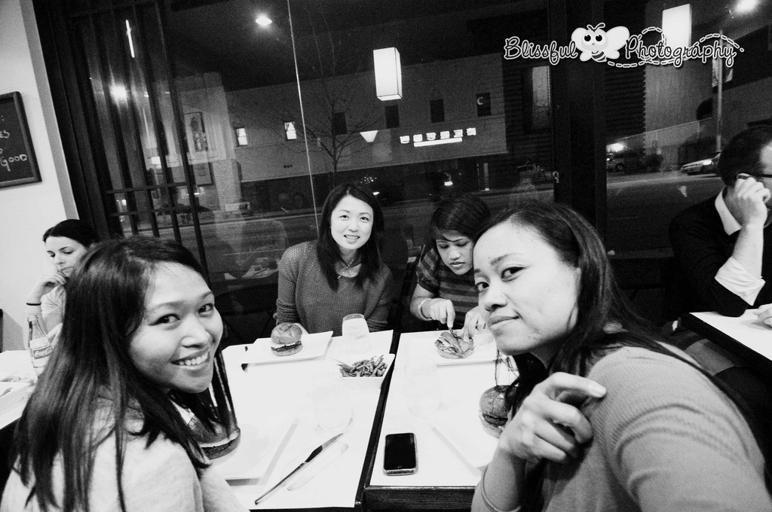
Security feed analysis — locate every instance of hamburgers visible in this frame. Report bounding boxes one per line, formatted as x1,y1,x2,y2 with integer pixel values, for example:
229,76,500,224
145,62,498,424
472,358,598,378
435,332,476,358
480,385,517,438
270,321,304,356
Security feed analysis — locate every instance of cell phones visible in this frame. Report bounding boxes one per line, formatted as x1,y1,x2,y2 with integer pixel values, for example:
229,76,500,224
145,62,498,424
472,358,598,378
384,433,416,476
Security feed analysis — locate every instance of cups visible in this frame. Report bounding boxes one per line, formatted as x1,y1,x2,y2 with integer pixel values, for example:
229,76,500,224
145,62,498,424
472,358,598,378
340,312,373,358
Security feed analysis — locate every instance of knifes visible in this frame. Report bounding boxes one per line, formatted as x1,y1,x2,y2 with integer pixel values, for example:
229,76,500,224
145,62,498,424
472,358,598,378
253,432,345,506
448,322,464,354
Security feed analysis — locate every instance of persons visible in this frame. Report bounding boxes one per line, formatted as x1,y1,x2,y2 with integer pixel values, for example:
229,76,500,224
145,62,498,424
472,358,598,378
275,179,396,336
0,234,249,512
239,204,289,261
407,193,494,330
24,218,102,379
469,198,772,512
665,122,772,432
186,205,257,317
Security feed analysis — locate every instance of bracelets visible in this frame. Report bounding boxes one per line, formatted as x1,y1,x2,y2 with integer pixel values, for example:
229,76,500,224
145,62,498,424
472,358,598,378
480,462,527,512
26,301,42,306
418,297,432,322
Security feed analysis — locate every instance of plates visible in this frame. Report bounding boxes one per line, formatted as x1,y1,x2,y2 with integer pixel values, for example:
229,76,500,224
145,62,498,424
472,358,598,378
428,408,507,469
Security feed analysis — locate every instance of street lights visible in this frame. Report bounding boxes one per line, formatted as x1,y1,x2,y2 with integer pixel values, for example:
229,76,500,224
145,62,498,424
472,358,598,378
710,1,760,154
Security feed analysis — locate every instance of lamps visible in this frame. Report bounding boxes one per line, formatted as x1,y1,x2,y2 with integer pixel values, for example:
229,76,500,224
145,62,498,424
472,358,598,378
373,47,403,103
360,130,378,144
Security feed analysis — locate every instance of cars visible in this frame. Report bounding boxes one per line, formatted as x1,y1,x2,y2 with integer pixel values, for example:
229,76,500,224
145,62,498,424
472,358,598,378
678,152,720,175
608,150,642,171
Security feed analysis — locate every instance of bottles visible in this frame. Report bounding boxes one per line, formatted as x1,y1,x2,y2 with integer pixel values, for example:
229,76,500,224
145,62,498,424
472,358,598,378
428,330,510,366
239,329,334,363
26,313,52,378
203,416,298,482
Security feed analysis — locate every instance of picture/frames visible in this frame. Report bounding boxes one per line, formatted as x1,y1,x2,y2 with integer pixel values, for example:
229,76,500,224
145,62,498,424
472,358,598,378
0,91,42,189
